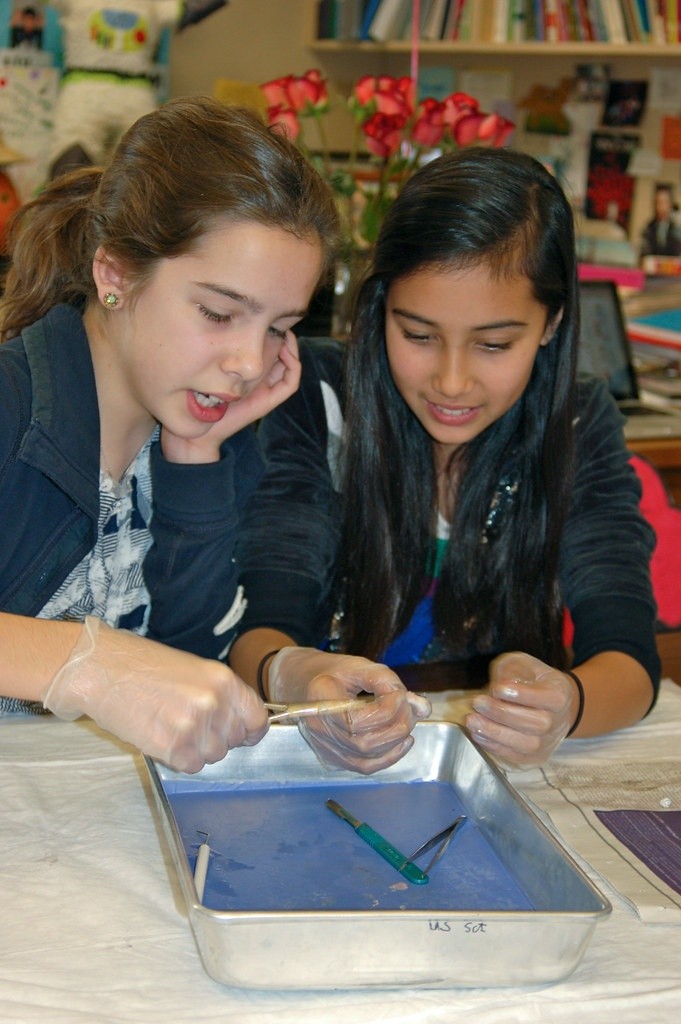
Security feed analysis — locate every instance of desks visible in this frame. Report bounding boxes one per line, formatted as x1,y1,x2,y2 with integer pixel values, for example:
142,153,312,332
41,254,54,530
0,680,681,1020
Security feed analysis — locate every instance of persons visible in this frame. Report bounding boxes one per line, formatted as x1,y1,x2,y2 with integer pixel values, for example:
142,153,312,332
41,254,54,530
233,145,661,775
39,1,227,188
0,97,340,772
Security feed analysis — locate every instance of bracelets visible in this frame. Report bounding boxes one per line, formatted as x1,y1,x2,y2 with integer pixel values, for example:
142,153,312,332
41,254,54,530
257,649,279,703
567,671,585,738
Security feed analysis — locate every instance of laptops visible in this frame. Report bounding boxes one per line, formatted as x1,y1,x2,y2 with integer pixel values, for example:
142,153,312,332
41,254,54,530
563,278,681,440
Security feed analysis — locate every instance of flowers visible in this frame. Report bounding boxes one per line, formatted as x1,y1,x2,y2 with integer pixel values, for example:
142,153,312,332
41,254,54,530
256,67,516,280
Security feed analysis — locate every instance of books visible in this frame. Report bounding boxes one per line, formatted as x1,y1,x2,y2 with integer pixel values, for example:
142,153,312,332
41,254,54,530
318,0,681,43
622,307,681,416
